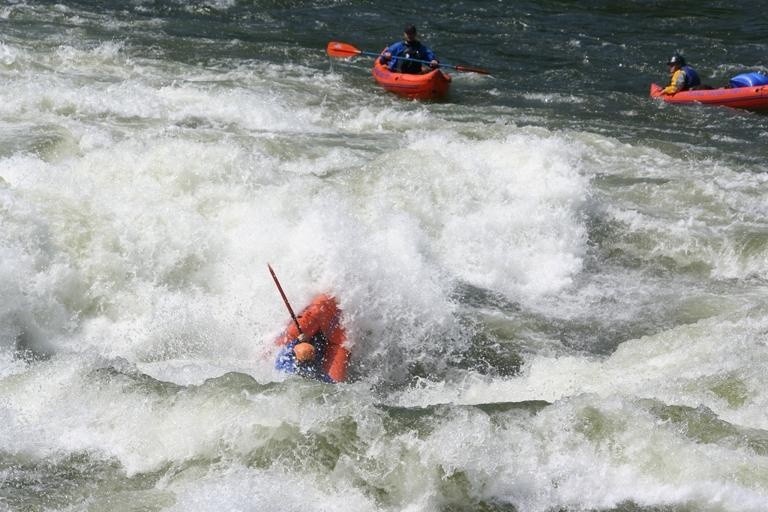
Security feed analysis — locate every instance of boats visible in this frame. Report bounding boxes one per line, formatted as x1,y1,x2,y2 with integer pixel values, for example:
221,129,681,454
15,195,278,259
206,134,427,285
271,291,353,384
373,49,452,101
648,81,768,110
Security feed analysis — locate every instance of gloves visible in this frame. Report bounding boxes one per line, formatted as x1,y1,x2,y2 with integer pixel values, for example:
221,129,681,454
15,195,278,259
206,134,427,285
297,333,306,342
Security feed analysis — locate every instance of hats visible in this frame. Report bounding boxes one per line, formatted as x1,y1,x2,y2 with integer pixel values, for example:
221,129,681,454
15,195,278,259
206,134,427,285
403,25,416,34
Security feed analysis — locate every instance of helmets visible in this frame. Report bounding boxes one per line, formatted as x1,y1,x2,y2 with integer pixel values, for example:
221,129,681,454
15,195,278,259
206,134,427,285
293,343,317,363
667,55,683,65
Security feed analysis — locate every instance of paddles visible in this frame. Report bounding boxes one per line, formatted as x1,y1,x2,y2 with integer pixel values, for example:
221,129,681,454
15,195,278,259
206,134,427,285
326,41,490,75
267,263,302,335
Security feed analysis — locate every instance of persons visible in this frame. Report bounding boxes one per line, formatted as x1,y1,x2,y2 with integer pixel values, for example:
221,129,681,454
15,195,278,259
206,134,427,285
274,333,334,385
379,24,439,74
660,55,699,94
